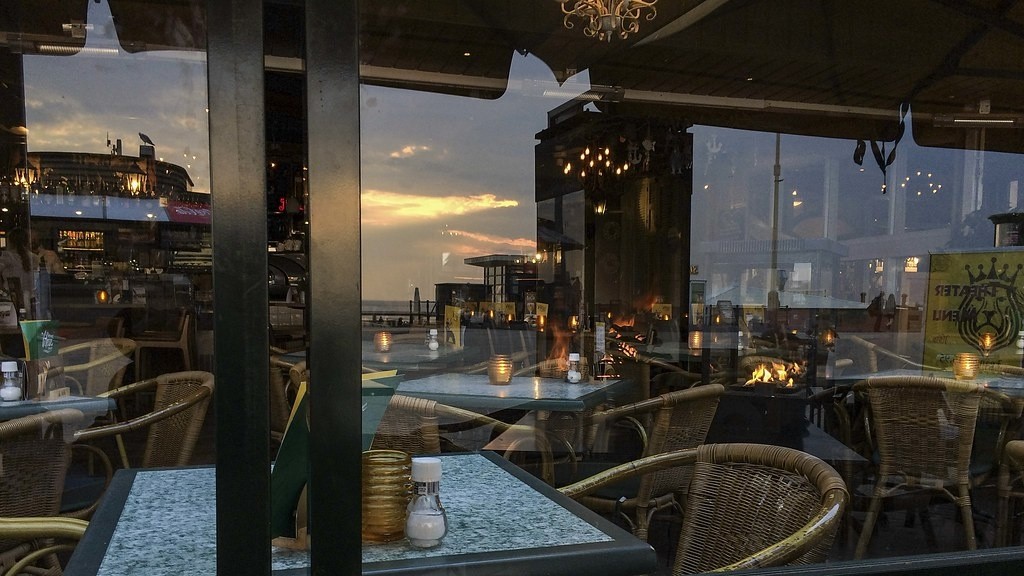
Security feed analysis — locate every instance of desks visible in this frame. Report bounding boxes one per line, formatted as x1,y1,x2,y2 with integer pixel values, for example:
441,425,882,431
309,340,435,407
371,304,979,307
59,450,659,575
1,390,117,427
394,374,625,476
825,368,1024,474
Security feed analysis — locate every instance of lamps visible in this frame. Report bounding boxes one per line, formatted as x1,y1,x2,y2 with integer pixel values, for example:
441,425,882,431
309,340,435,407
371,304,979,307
563,135,638,216
558,0,658,43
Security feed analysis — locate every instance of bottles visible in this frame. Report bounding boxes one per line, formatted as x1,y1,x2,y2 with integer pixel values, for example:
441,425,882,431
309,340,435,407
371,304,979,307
69,251,74,268
568,354,581,383
63,251,69,268
59,231,103,248
403,458,446,550
74,252,78,264
0,362,23,402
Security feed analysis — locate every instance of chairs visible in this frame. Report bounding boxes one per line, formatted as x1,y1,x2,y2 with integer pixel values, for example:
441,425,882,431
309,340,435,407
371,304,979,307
554,443,851,576
266,328,727,543
0,302,217,576
728,325,1024,564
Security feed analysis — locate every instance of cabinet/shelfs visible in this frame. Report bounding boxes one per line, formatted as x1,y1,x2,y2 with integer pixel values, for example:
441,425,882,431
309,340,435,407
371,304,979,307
50,226,118,275
